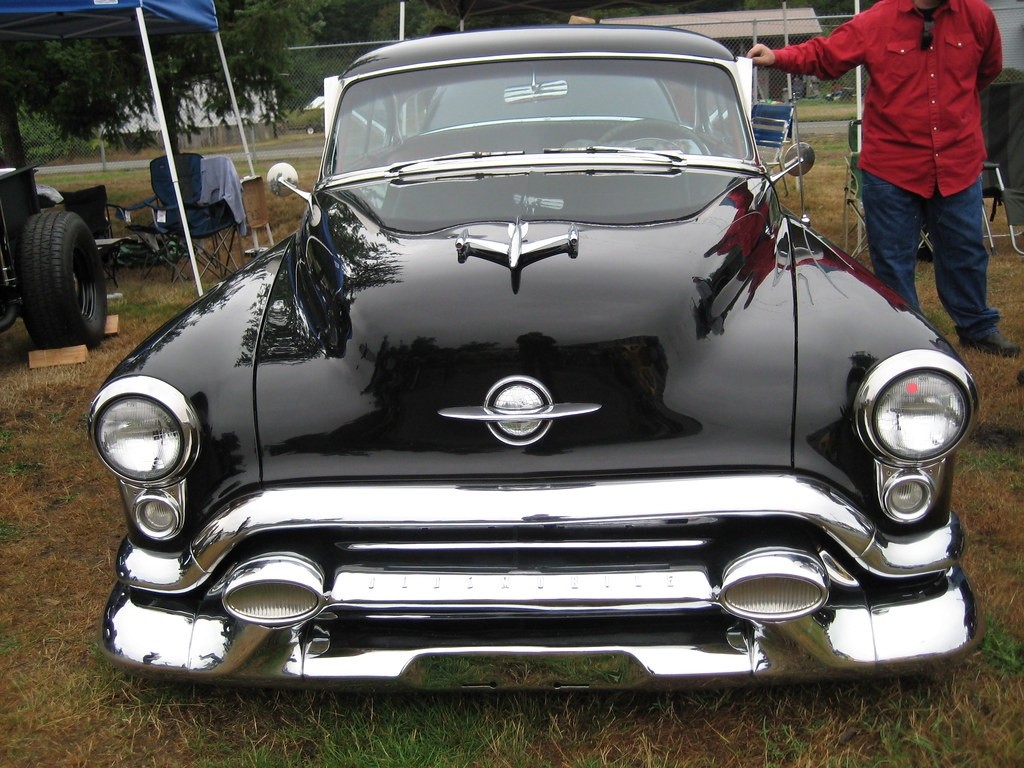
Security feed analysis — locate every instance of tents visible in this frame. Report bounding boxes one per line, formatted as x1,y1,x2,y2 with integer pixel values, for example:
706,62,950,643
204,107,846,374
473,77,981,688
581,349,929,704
0,0,275,297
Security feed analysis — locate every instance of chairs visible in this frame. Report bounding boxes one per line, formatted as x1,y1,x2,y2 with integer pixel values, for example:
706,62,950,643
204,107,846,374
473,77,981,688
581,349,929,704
241,178,273,264
117,153,203,283
59,184,137,289
752,103,794,197
843,119,935,256
170,157,248,290
977,82,1024,255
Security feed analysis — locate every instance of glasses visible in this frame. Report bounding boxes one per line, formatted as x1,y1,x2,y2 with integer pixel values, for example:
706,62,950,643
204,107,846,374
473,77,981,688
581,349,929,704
920,13,935,50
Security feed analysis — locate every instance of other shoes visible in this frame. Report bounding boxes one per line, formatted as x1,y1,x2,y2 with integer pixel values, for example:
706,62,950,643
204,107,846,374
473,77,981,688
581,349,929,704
959,331,1021,358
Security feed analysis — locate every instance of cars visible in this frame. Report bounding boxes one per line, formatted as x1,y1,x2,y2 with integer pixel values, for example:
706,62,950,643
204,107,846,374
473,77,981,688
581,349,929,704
82,20,981,700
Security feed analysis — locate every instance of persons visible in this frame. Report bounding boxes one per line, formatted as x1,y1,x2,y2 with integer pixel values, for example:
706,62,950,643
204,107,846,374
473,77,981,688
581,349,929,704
746,0,1021,357
